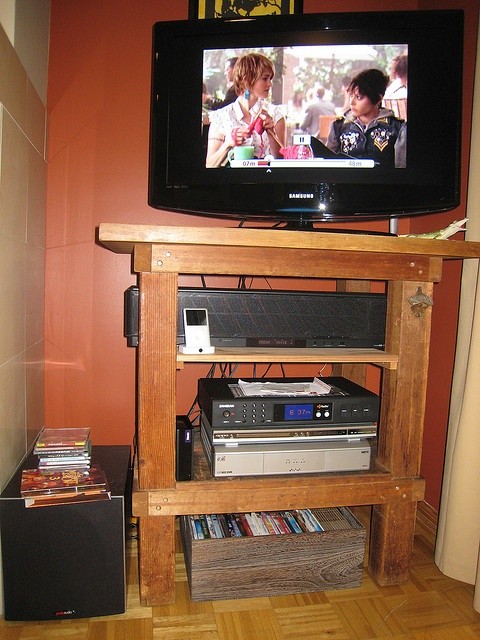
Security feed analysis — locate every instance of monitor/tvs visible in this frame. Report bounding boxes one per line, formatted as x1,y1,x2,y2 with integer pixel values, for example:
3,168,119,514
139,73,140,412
148,9,465,237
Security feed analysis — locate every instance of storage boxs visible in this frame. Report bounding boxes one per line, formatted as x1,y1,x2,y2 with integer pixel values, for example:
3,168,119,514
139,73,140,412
178,507,366,601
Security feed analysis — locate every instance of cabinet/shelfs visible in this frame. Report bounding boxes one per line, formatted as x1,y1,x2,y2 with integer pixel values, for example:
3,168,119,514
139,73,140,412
95,221,480,609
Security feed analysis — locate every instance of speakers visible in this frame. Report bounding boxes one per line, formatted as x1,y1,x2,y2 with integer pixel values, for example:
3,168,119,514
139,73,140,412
123,285,388,350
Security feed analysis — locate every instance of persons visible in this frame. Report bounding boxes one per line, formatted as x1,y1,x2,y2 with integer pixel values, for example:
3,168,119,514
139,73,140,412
391,54,408,97
332,76,353,109
218,56,241,108
206,53,285,167
325,69,406,167
300,88,336,137
286,87,309,124
202,84,220,108
201,110,211,163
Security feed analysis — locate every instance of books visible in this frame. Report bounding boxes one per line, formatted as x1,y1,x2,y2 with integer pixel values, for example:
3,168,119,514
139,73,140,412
311,135,381,158
21,425,110,509
188,507,361,542
